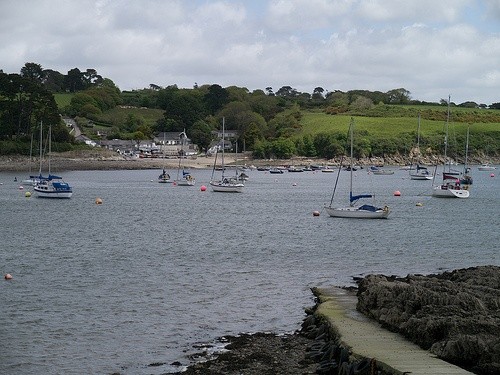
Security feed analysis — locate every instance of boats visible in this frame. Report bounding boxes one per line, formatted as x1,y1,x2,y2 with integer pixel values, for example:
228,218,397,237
477,164,496,171
432,179,470,199
215,163,395,175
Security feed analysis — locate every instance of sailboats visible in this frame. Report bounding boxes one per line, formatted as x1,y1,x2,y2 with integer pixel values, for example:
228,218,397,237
408,113,434,180
209,117,244,192
440,94,473,185
21,120,59,186
173,127,196,186
232,137,250,183
324,116,391,219
157,132,172,183
33,124,73,198
